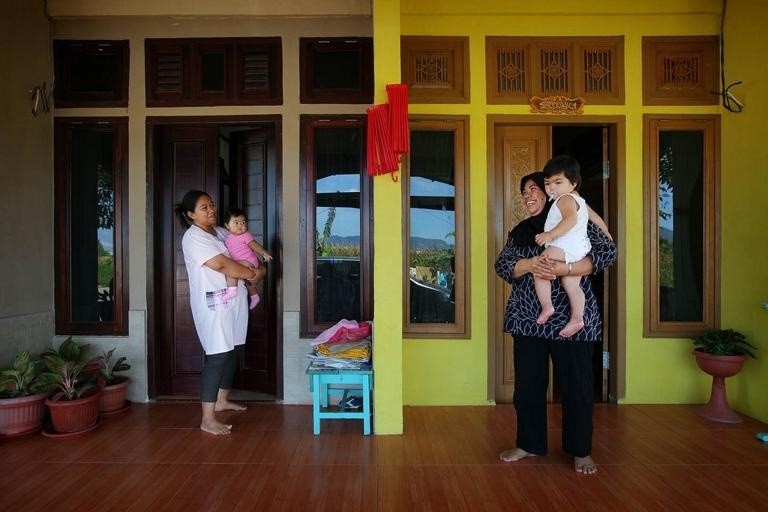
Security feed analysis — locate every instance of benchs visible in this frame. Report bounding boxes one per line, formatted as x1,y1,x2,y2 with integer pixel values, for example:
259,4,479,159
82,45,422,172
305,357,373,436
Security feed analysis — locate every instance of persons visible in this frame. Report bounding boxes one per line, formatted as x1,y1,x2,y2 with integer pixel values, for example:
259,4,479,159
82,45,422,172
175,190,266,435
223,208,272,309
534,154,613,337
494,171,617,474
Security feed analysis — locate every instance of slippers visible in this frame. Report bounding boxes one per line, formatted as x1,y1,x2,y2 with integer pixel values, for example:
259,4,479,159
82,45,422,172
338,399,360,410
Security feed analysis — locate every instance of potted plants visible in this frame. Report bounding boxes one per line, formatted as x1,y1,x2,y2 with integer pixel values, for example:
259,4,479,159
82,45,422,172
41,336,105,440
691,328,757,424
0,352,49,438
98,348,133,418
